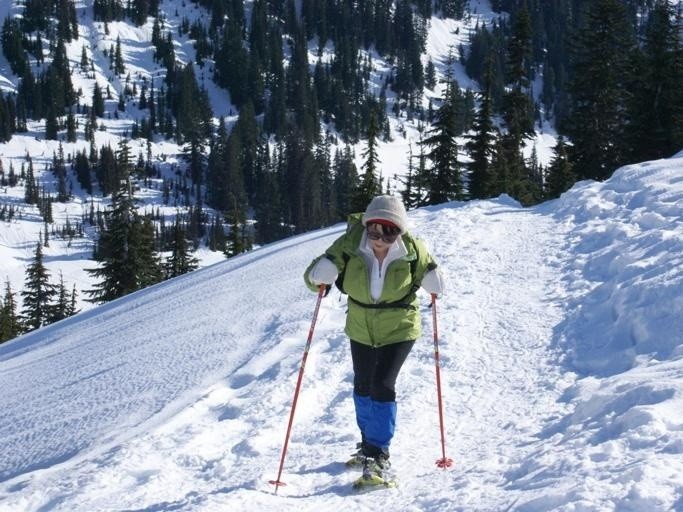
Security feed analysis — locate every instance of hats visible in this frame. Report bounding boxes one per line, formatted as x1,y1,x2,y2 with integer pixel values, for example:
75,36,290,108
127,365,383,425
361,195,406,234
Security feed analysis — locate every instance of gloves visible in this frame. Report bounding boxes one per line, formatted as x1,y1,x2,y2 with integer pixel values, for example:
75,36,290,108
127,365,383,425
421,269,445,293
309,258,339,285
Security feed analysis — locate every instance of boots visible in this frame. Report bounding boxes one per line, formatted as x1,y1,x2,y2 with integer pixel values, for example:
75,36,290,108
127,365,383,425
344,441,400,490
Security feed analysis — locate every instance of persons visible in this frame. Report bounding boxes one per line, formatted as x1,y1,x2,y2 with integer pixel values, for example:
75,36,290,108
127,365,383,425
302,193,448,487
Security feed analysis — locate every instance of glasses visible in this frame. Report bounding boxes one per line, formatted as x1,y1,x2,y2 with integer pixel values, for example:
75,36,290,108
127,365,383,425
366,229,396,243
